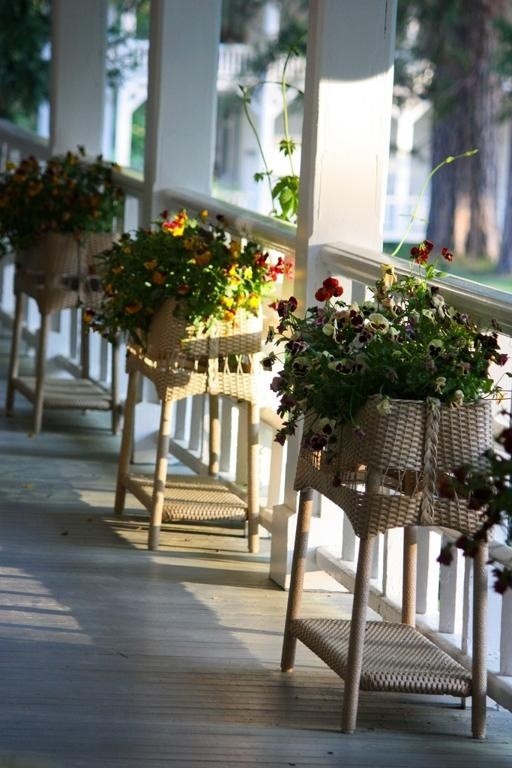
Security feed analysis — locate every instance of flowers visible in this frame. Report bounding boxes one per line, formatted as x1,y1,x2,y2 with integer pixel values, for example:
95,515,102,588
4,144,124,440
83,218,274,556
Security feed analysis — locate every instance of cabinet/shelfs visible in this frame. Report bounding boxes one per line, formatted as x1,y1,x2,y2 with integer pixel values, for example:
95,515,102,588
274,397,494,738
